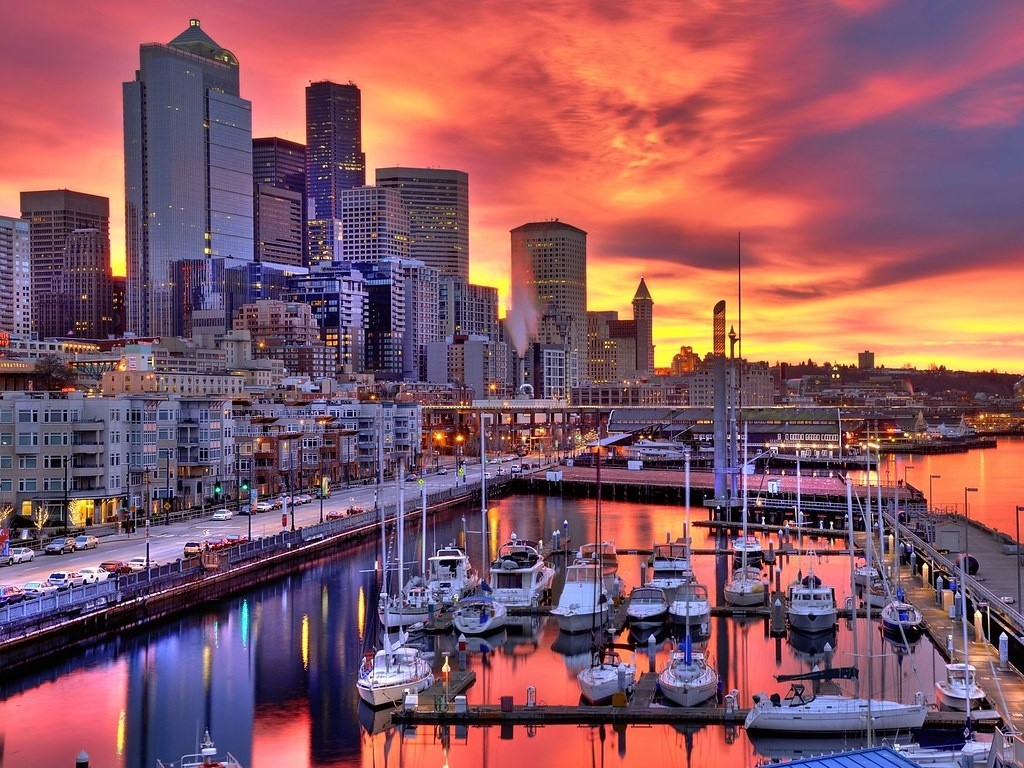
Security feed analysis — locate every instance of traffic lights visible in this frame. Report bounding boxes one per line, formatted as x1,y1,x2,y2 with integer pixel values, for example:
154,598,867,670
243,480,248,490
215,482,220,493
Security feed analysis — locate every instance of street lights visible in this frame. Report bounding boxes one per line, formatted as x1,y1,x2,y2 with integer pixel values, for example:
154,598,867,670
455,436,463,488
436,434,442,471
930,475,940,548
964,488,978,576
1016,507,1024,614
904,466,914,528
886,460,897,513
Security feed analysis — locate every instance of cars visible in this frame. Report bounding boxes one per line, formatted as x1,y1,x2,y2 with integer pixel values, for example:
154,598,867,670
212,508,232,521
513,454,519,459
437,468,448,475
239,487,331,514
506,455,513,460
511,465,521,472
490,458,497,464
326,511,343,520
495,468,506,476
481,473,492,481
0,556,158,607
184,534,249,557
43,535,99,554
531,462,540,469
521,462,530,471
405,474,417,482
0,547,35,567
499,456,508,463
347,506,363,515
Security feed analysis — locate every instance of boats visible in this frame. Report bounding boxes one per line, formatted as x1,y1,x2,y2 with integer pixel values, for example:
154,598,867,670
156,729,241,768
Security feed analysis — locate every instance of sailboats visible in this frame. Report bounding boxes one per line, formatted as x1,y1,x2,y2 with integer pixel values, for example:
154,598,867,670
351,390,1017,767
